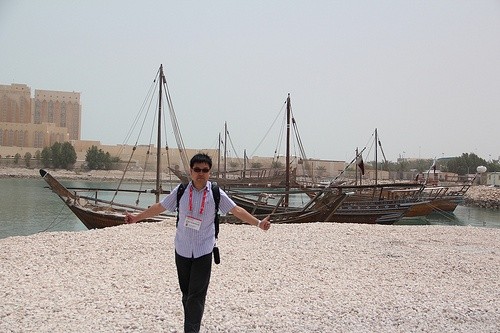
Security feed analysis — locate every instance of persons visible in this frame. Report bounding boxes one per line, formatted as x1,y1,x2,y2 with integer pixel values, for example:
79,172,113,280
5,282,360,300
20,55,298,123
125,154,271,333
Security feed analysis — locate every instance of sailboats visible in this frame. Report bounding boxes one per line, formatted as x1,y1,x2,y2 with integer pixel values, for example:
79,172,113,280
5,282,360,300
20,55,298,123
167,91,479,226
38,62,347,231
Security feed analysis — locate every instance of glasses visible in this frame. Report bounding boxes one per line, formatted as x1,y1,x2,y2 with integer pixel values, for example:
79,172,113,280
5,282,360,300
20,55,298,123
192,168,211,173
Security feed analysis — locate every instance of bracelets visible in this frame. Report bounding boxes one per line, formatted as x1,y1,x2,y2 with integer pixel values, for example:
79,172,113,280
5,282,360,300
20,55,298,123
257,220,261,227
